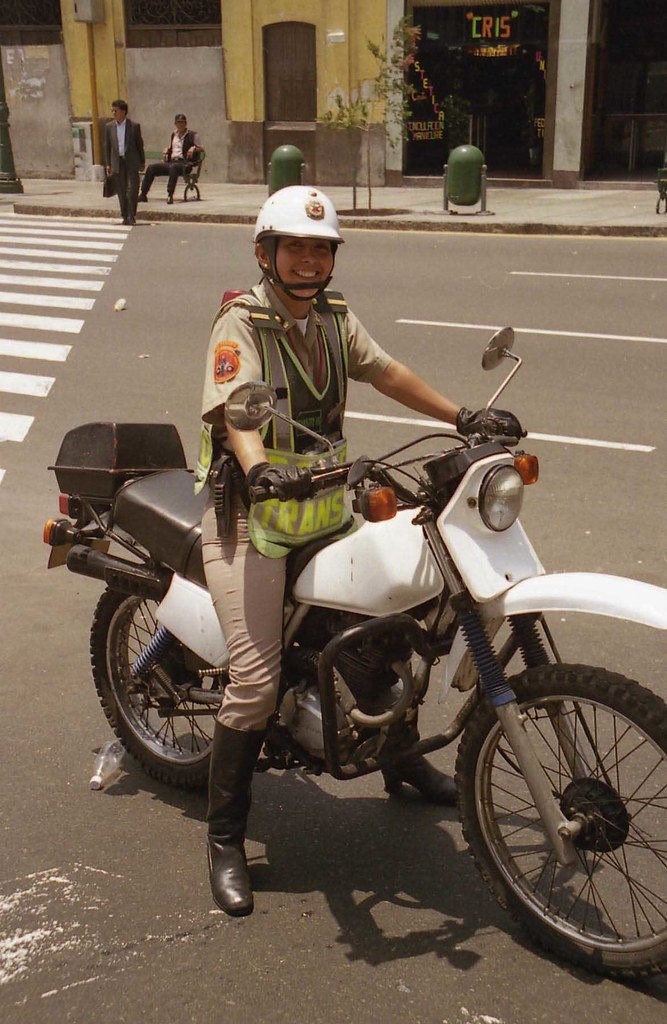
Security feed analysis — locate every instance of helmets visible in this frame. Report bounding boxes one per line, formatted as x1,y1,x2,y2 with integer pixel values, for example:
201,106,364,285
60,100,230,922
254,185,345,253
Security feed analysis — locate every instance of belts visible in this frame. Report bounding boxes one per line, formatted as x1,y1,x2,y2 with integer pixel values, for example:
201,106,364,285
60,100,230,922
119,156,125,159
173,158,183,160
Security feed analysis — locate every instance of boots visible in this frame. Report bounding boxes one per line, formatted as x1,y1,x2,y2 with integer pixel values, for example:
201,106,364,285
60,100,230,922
373,677,456,807
206,717,270,917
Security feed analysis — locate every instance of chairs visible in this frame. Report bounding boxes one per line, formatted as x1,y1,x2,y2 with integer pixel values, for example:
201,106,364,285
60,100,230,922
653,156,667,214
180,149,206,202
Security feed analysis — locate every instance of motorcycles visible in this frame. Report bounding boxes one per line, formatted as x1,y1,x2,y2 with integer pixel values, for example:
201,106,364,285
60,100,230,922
43,325,667,984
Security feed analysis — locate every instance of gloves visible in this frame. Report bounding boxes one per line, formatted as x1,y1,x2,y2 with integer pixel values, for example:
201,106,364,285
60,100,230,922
245,461,313,503
456,407,522,446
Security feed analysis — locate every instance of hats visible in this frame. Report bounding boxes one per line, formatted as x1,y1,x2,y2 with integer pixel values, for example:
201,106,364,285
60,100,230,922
175,114,187,123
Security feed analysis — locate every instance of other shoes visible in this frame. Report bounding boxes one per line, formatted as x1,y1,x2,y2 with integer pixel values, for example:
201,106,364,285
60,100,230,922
167,196,173,204
137,195,148,202
123,216,136,225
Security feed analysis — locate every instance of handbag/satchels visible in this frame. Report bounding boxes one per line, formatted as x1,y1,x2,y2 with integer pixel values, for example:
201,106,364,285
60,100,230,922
102,177,115,197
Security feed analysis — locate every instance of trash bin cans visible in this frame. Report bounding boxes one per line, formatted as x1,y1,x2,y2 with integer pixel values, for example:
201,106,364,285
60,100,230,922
267,145,307,196
443,144,488,211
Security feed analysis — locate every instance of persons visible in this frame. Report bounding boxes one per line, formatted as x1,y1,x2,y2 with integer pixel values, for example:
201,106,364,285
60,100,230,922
103,100,145,225
193,186,522,914
138,114,204,204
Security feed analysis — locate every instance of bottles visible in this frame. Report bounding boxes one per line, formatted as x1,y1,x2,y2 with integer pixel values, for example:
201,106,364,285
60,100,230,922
89,741,126,790
